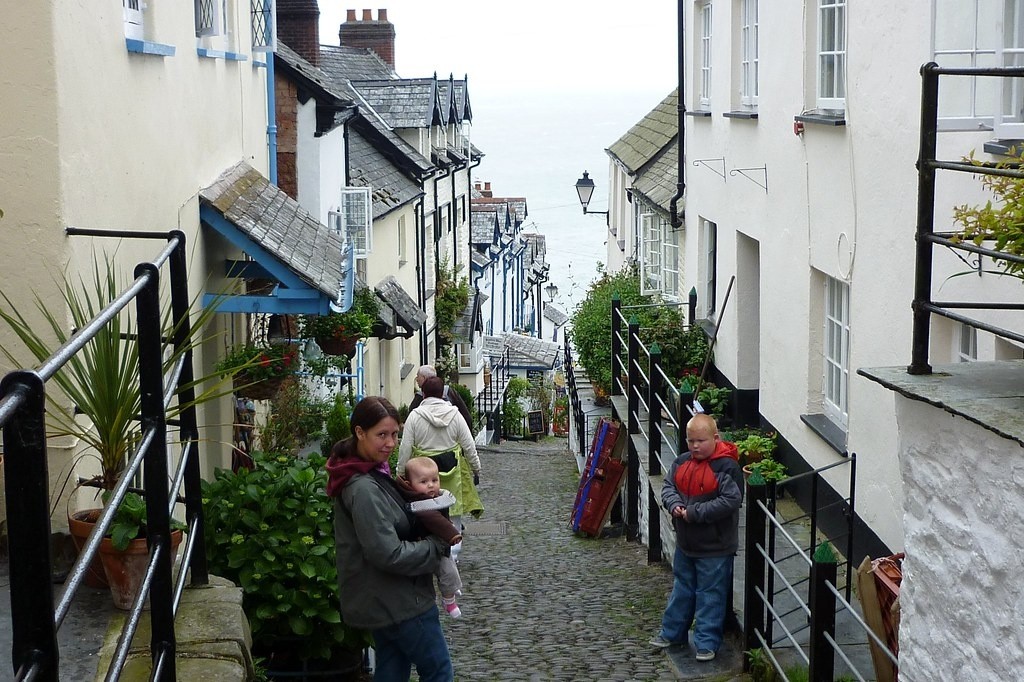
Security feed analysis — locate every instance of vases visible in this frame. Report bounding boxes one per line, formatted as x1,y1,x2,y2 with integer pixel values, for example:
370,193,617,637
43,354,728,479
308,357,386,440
313,336,361,355
232,373,288,398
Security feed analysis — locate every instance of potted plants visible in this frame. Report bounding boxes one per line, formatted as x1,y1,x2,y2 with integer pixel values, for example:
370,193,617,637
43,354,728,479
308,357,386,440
0,224,289,616
484,360,493,386
565,258,735,432
724,424,789,497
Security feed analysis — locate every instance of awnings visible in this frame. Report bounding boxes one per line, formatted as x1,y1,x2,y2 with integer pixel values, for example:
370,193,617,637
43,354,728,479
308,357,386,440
352,274,394,336
197,161,353,316
376,276,428,331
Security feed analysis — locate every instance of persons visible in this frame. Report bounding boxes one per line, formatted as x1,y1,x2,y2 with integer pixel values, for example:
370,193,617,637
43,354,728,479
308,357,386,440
397,457,464,618
645,413,744,660
408,365,473,438
396,377,484,559
323,395,453,682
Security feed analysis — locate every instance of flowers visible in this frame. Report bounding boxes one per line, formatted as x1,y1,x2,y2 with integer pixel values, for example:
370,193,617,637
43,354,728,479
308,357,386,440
292,306,374,344
211,340,301,380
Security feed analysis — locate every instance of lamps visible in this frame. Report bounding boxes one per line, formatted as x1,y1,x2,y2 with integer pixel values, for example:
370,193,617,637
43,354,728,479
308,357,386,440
574,170,609,226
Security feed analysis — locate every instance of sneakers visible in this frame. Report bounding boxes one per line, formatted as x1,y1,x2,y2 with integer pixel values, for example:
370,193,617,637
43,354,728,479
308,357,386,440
649,636,674,647
696,649,715,660
